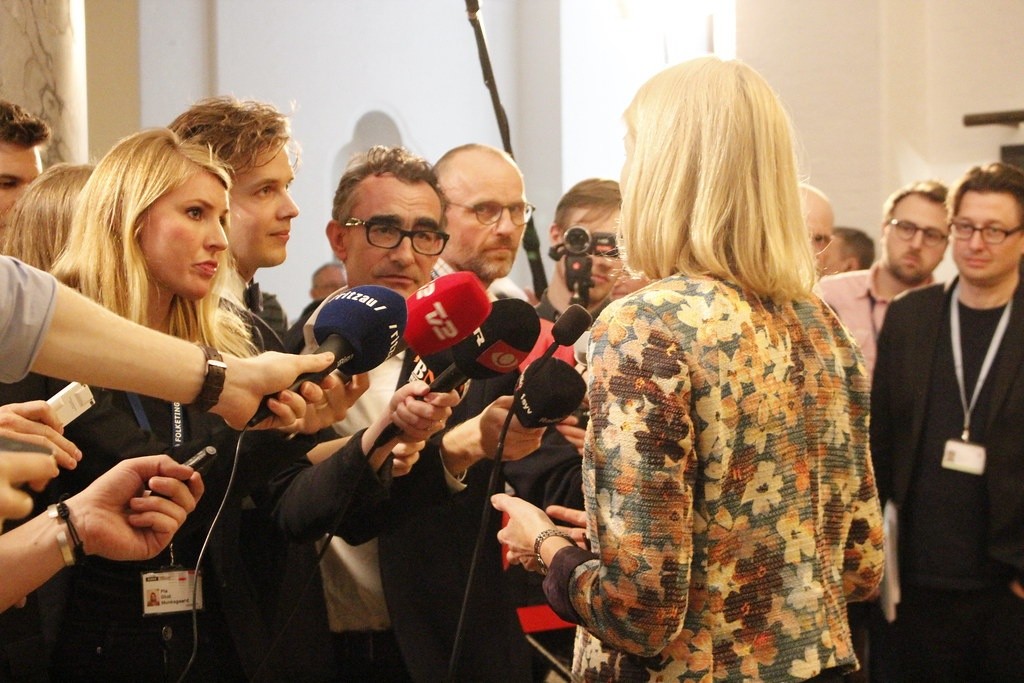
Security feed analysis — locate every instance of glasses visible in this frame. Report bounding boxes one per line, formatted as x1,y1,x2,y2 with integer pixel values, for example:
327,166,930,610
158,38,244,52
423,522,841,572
812,235,832,255
948,223,1024,243
889,219,946,245
447,201,534,226
343,216,449,255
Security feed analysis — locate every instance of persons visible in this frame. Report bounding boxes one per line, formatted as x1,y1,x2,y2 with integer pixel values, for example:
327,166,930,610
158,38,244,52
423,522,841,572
814,226,874,284
791,186,835,290
0,99,53,251
3,163,99,293
278,144,653,683
0,254,334,430
811,180,951,396
491,57,885,683
147,590,160,606
868,163,1024,683
0,400,82,535
0,127,459,683
0,454,205,615
162,97,547,683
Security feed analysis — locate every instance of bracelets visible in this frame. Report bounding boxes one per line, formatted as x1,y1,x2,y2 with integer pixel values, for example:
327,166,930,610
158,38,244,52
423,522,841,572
57,501,81,547
48,504,74,566
535,530,579,577
189,346,227,412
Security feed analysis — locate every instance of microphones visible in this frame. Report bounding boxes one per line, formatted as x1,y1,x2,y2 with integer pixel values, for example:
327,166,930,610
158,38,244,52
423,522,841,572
375,298,541,449
327,273,490,385
513,357,588,428
249,286,407,428
552,304,592,346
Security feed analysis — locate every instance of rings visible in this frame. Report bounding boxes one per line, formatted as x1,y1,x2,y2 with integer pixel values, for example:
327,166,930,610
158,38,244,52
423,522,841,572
425,422,434,431
316,401,330,409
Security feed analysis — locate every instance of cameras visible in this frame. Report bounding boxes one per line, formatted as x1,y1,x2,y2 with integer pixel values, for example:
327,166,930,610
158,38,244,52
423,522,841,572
563,227,620,258
